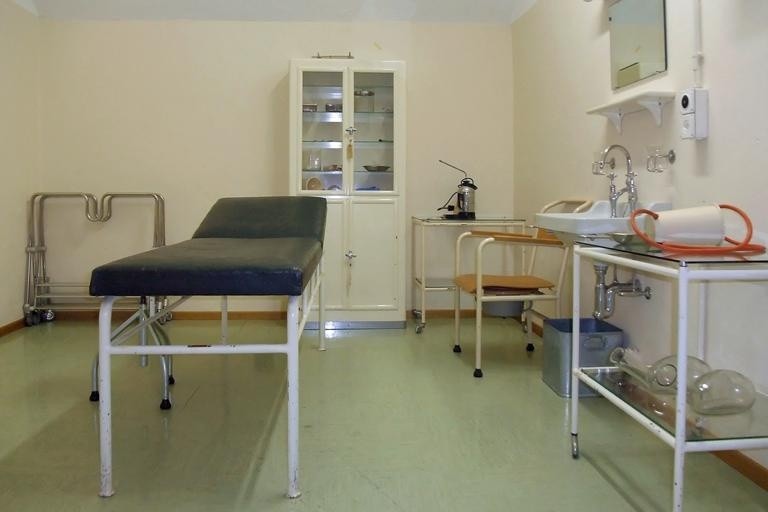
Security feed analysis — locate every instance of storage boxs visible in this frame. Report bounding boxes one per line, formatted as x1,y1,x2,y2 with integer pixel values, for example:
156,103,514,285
541,317,625,399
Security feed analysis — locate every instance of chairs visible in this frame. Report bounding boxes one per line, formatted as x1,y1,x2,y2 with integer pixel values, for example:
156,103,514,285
452,199,593,377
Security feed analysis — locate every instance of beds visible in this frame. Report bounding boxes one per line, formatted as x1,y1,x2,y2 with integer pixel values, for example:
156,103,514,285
89,196,327,499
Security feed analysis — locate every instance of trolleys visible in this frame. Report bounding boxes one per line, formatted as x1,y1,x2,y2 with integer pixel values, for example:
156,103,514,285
20,188,175,327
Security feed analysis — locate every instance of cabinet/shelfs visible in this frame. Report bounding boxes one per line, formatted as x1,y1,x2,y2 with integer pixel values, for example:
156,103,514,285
287,59,408,331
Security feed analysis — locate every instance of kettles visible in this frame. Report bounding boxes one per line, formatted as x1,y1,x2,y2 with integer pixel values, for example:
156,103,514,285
454,180,478,220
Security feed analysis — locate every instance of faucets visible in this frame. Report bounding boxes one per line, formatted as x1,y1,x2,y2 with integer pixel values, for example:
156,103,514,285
592,145,638,216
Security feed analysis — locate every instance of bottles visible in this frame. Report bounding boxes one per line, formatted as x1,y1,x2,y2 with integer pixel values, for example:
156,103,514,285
353,89,375,112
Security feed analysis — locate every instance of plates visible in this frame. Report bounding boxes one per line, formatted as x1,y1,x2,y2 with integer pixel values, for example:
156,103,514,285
362,165,391,172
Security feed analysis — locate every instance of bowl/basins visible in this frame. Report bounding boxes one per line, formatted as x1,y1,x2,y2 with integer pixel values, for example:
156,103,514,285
305,176,323,190
327,184,340,190
605,231,647,245
323,165,338,172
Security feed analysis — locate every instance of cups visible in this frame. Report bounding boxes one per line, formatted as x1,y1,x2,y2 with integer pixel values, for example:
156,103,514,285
309,154,322,172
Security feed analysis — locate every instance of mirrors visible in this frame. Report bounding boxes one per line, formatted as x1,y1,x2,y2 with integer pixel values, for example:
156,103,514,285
606,0,667,90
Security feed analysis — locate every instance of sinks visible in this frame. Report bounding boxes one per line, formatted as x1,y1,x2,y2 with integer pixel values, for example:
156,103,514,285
534,201,670,236
